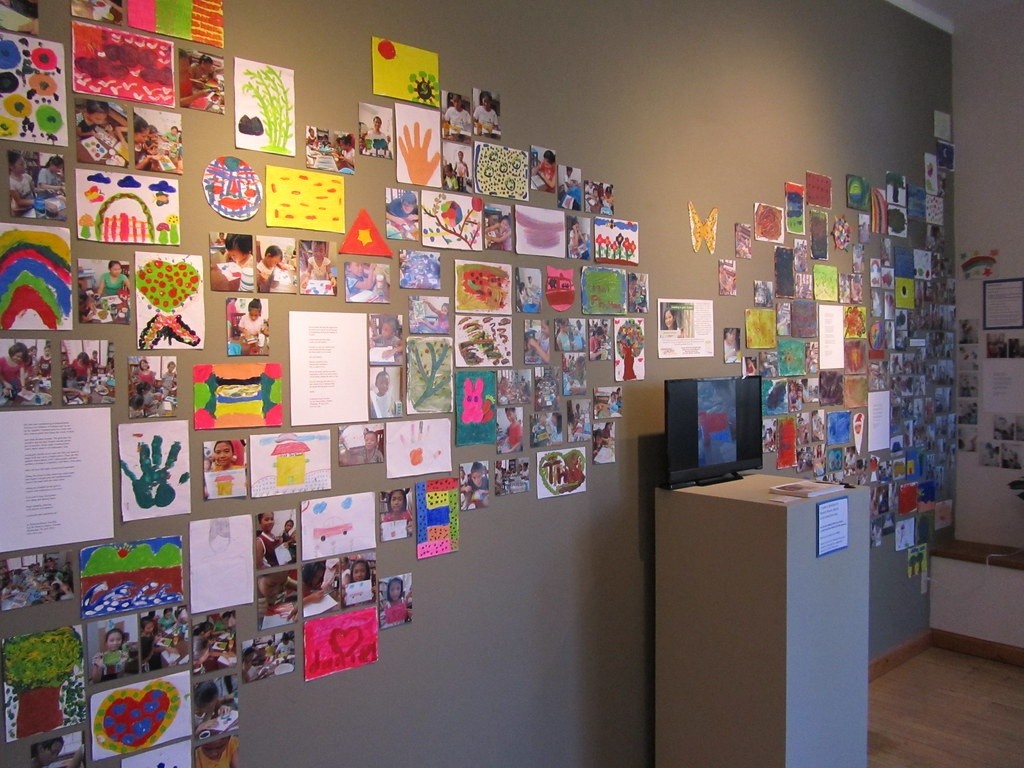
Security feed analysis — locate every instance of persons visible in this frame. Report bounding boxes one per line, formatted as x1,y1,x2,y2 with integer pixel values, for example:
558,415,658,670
243,632,295,682
0,558,74,600
0,342,52,393
177,47,216,104
0,0,122,25
194,680,238,735
204,442,247,496
77,260,129,322
76,99,129,148
92,630,137,683
193,612,235,666
129,359,178,414
31,737,64,768
62,351,114,398
8,154,65,217
133,115,182,169
210,233,254,292
227,94,1024,620
139,606,188,671
195,735,239,768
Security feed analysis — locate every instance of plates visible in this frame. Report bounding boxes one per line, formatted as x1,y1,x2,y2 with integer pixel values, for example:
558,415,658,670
212,642,227,651
206,710,238,732
275,663,294,675
156,638,172,647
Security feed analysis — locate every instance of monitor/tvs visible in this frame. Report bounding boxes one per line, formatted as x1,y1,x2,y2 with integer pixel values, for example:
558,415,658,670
665,374,763,490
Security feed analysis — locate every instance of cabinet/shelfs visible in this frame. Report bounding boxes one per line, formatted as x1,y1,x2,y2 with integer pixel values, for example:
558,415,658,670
928,540,1024,667
654,473,870,768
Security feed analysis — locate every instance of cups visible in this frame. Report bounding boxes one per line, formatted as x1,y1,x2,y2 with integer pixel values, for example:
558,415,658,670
199,731,210,740
